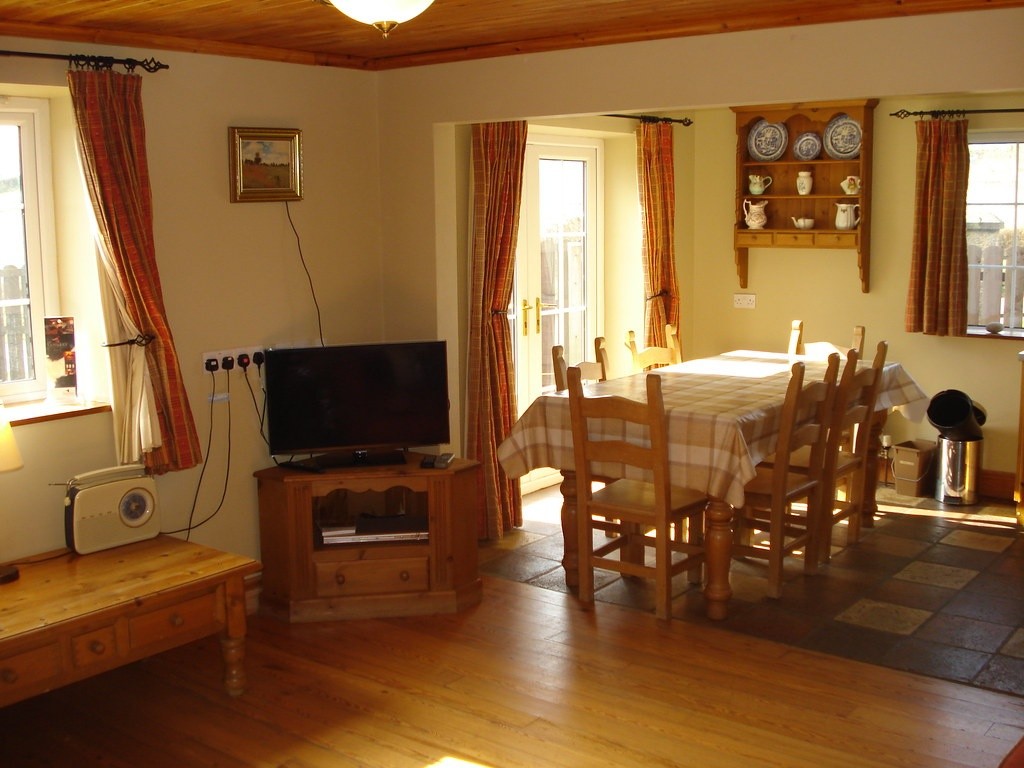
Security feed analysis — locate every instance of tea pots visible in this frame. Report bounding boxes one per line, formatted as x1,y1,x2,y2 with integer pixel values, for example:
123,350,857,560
790,216,815,230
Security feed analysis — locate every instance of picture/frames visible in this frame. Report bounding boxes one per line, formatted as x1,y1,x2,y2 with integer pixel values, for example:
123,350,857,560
227,126,304,204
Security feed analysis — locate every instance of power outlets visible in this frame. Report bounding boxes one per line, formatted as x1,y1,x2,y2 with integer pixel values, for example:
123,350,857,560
252,345,265,366
235,347,252,370
220,348,236,371
202,351,222,374
733,294,756,310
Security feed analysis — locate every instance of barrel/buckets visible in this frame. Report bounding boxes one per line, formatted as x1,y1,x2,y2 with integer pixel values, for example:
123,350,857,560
926,388,983,442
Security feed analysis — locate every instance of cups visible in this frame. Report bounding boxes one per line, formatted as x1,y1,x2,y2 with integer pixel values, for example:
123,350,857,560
847,175,861,195
748,175,772,195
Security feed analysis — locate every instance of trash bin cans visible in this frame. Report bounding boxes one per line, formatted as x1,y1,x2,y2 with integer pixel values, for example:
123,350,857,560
926,389,987,505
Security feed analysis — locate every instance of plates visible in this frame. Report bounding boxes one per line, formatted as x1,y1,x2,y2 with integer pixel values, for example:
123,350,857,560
747,119,789,162
823,115,862,160
792,132,822,161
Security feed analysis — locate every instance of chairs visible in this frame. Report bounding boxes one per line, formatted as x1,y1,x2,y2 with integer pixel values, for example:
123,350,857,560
625,324,682,373
728,352,842,589
742,340,889,562
552,336,687,545
787,319,865,362
566,365,711,622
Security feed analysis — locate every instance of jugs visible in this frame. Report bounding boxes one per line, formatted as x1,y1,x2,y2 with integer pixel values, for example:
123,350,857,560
834,202,862,230
742,199,768,230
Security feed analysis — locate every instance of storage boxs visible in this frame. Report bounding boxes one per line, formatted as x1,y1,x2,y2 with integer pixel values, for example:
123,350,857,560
890,438,937,498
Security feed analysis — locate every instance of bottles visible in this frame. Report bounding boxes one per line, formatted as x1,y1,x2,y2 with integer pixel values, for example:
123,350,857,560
796,171,813,195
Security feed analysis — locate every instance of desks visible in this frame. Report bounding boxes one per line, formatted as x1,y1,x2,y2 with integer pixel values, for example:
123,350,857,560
495,348,933,623
0,532,266,711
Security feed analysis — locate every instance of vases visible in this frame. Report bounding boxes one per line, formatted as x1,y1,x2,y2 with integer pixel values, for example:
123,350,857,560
796,171,815,196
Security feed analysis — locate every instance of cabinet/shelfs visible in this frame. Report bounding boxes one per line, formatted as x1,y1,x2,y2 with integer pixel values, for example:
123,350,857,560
252,451,484,626
728,97,880,295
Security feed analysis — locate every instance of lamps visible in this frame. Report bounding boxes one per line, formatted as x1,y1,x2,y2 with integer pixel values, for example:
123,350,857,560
0,398,24,584
321,0,435,39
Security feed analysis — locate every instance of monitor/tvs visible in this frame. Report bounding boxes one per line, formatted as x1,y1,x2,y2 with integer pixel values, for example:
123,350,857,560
263,340,449,472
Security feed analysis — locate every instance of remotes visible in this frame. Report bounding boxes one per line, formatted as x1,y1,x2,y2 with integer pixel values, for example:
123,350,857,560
434,453,455,469
420,455,436,468
